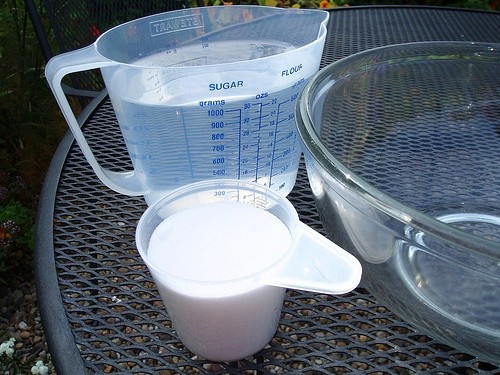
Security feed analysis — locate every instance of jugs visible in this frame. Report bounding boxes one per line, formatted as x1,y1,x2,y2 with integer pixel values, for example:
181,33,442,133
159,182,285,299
45,4,331,219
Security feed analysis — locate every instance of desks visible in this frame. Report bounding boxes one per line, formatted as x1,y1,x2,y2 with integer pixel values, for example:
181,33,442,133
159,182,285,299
34,5,500,375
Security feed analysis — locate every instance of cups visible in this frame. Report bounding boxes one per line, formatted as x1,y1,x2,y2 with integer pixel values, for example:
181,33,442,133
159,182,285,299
136,179,362,361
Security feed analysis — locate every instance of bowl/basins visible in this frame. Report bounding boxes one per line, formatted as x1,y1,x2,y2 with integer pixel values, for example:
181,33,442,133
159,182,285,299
296,42,500,364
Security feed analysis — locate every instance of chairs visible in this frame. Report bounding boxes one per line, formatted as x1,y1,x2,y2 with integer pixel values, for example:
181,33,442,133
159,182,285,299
26,0,212,109
